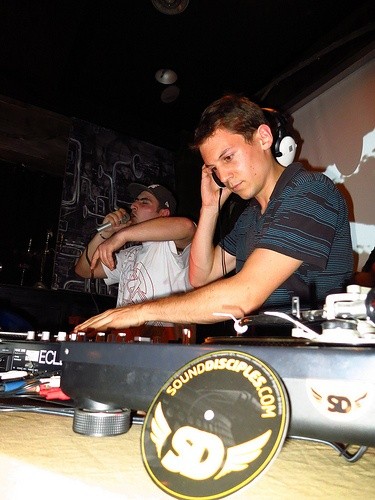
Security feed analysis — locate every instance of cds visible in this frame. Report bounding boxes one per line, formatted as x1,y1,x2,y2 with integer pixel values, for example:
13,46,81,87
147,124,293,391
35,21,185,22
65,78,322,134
140,349,290,500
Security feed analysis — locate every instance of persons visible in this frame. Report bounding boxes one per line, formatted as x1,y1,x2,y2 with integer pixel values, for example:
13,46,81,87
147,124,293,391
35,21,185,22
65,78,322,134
73,96,355,333
75,186,197,327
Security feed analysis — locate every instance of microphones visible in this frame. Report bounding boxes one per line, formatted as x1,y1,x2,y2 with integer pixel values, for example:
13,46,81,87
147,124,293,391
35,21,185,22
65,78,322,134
92,213,130,233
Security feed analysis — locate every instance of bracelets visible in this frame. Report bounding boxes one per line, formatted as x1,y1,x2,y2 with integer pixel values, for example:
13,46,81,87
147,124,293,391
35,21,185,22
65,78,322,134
99,231,108,240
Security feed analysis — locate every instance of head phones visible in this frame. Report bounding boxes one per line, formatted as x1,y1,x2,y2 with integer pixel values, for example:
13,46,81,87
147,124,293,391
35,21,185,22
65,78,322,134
210,108,297,188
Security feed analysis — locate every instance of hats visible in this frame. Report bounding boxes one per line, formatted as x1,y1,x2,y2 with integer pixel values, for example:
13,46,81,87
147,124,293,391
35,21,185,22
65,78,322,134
127,183,177,215
